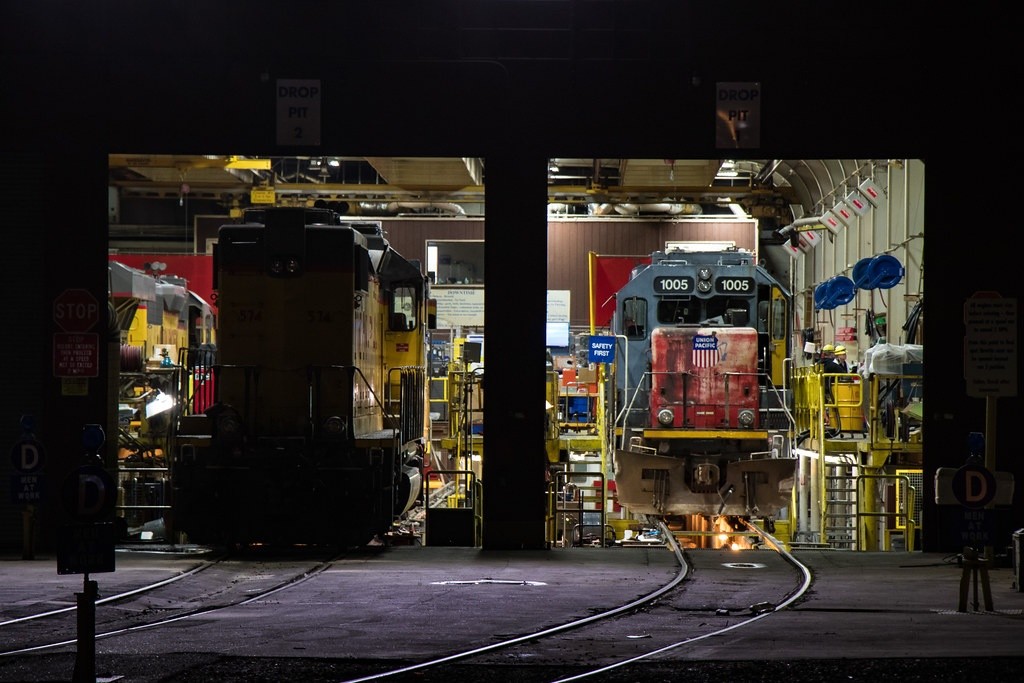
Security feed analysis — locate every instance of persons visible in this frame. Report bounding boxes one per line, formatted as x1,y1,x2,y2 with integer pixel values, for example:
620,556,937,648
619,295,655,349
821,344,851,404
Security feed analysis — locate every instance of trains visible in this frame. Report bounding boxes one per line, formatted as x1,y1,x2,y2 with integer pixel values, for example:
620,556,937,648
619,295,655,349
108,205,605,550
611,248,799,529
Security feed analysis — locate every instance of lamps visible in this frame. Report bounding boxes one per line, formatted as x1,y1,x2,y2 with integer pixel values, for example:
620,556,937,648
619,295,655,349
783,239,803,260
856,177,888,209
830,201,858,227
818,210,845,236
798,230,822,254
548,161,560,173
843,191,871,217
